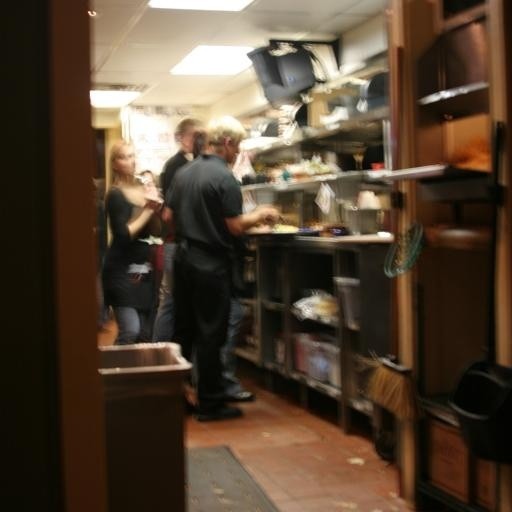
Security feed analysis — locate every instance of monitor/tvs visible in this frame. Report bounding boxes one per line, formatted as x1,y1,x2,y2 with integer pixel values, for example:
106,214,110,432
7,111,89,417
247,44,316,107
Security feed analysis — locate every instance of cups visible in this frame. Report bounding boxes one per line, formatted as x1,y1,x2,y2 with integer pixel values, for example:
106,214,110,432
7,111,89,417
358,209,384,235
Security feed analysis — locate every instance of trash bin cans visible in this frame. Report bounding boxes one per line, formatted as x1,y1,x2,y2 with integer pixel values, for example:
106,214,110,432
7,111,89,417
99,341,192,511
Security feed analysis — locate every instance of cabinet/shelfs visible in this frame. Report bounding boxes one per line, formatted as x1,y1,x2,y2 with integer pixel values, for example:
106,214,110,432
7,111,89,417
228,223,394,435
237,117,389,193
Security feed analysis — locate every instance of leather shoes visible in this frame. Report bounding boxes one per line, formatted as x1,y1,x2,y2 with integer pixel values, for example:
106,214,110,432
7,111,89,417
226,391,253,401
198,406,243,421
